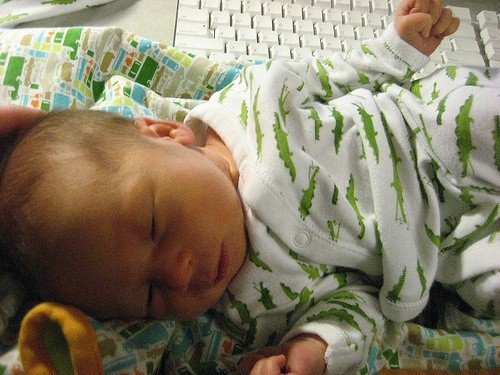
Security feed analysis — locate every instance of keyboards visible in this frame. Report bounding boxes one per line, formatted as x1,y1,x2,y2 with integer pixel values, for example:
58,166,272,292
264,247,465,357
172,0,500,69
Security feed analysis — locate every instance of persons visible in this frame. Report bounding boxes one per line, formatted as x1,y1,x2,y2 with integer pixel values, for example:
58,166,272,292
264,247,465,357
0,1,500,375
